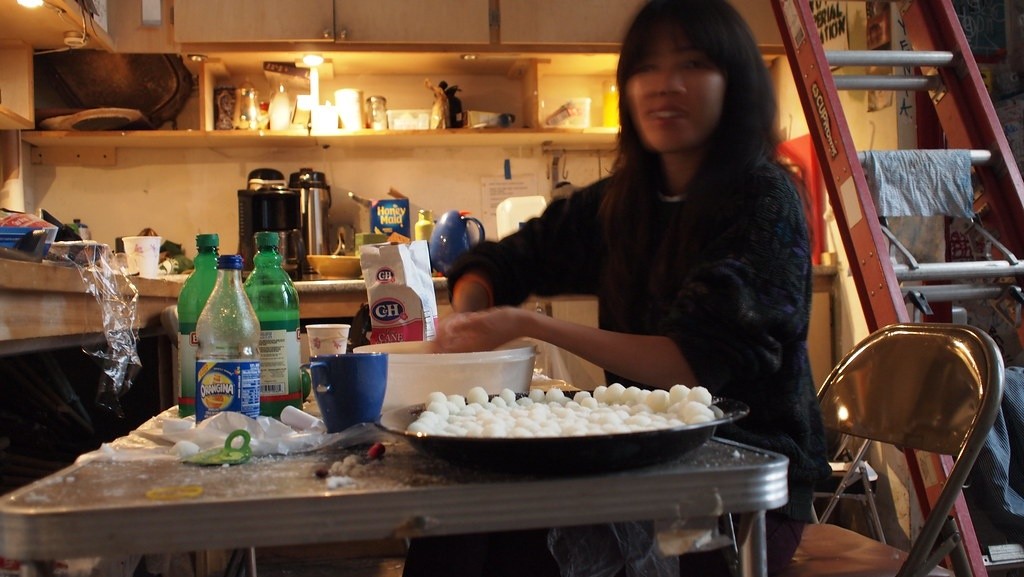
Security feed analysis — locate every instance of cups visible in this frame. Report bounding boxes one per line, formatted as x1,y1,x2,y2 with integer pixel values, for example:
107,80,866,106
566,98,591,130
308,352,388,432
121,236,162,279
304,324,351,392
602,79,619,129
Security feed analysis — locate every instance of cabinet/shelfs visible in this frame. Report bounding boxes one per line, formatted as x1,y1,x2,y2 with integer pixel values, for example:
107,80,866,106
0,0,836,449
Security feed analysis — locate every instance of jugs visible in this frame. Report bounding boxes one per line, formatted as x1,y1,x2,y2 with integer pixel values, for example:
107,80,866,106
289,168,332,273
254,226,306,280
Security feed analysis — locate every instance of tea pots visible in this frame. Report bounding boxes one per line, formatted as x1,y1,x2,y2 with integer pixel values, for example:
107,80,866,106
218,76,277,132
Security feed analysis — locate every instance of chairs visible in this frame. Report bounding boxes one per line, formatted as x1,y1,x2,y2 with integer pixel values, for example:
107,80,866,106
665,323,1006,577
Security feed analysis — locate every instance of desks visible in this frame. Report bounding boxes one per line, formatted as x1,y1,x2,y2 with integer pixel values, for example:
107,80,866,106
0,382,790,577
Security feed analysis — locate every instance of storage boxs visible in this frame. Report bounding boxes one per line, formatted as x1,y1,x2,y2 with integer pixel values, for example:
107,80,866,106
387,109,432,130
348,187,411,244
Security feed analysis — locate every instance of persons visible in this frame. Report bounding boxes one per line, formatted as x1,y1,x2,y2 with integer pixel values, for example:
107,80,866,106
400,0,835,577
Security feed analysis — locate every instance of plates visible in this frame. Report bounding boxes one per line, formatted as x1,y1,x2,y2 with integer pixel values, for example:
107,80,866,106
373,389,752,467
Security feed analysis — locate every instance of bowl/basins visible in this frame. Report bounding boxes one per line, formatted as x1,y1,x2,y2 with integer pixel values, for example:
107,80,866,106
44,242,109,266
352,339,542,415
303,251,362,280
0,226,58,256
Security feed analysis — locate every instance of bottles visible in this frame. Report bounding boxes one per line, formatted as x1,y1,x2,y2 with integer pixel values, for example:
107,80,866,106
66,218,90,240
243,232,311,417
177,233,224,418
195,254,261,425
414,209,435,240
333,88,389,131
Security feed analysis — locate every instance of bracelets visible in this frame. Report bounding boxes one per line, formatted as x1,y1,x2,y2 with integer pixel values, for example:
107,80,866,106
453,273,493,308
